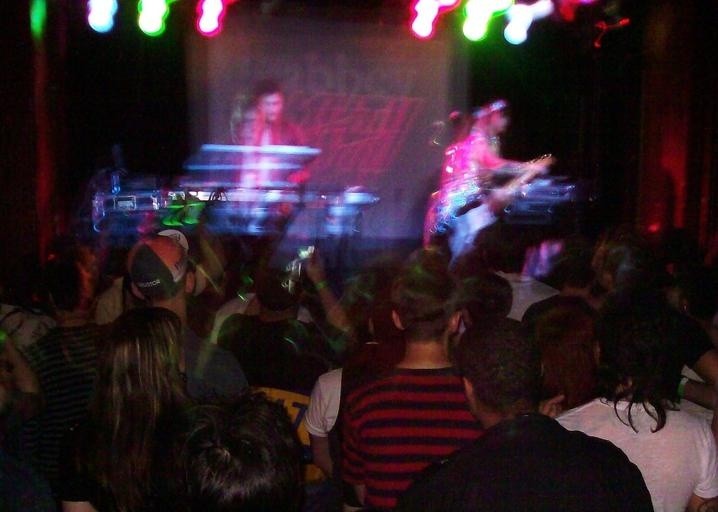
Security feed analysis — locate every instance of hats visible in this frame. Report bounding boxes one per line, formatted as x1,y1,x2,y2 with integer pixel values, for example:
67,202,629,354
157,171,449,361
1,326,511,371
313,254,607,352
126,227,192,298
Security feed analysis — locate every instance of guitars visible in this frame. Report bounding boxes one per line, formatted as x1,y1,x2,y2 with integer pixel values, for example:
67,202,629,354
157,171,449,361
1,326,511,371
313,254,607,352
428,153,557,275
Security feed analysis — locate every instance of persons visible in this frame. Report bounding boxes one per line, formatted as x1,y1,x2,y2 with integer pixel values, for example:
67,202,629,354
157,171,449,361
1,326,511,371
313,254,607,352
424,85,555,247
203,77,311,250
